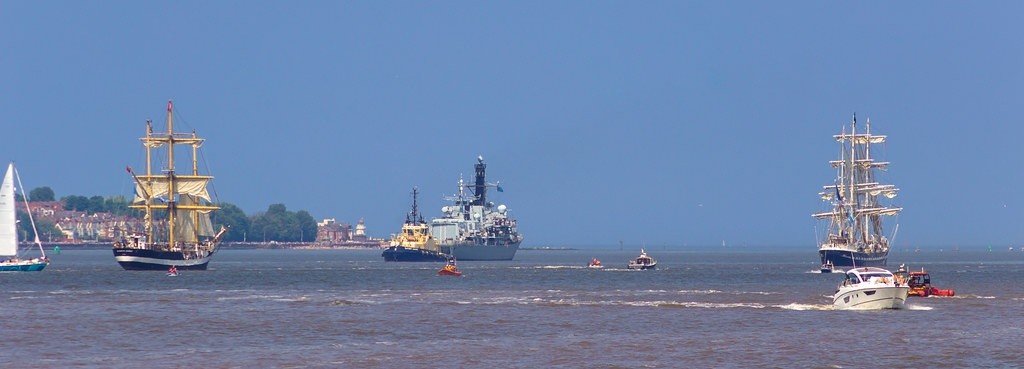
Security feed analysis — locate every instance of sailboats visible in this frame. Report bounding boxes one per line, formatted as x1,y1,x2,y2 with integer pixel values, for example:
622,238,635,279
0,160,52,273
112,97,232,271
809,111,905,275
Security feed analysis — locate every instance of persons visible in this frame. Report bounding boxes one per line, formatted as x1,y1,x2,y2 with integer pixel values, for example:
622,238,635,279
444,264,452,270
875,272,912,288
0,256,49,263
116,236,133,249
169,266,177,273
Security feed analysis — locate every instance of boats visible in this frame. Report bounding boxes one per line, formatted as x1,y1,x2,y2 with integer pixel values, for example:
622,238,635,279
586,263,605,270
892,262,955,298
627,240,657,270
832,249,911,312
438,257,463,277
381,186,456,263
166,265,183,278
428,155,524,261
